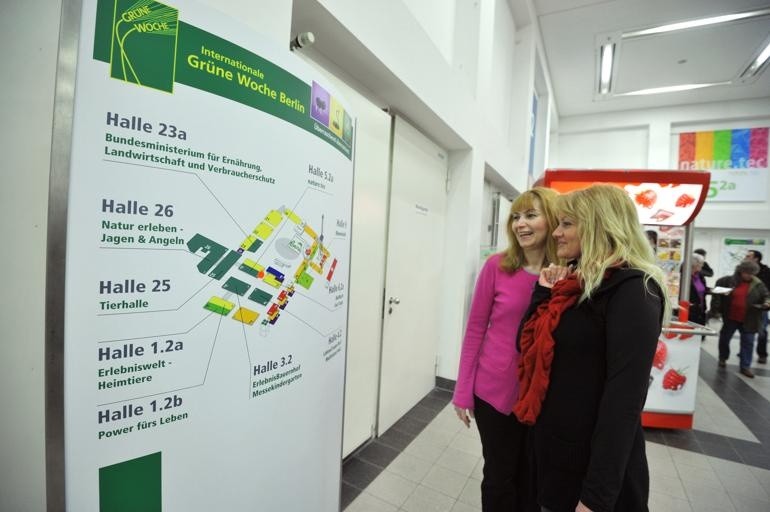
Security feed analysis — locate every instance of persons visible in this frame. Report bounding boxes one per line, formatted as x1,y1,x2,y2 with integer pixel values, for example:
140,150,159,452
736,250,770,363
677,254,706,340
515,184,669,512
711,259,770,378
452,188,567,512
694,250,713,293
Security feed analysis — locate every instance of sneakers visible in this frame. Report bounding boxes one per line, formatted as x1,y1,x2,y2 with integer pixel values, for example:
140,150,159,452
719,359,726,367
759,357,766,363
740,367,754,377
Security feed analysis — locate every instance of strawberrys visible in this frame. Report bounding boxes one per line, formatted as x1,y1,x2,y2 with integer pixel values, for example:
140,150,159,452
675,194,694,207
651,341,667,370
662,366,689,391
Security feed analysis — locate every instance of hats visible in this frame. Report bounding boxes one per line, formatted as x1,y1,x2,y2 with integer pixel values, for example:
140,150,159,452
739,261,759,274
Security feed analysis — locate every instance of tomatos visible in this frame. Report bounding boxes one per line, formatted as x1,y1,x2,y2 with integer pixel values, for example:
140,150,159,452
634,189,657,208
